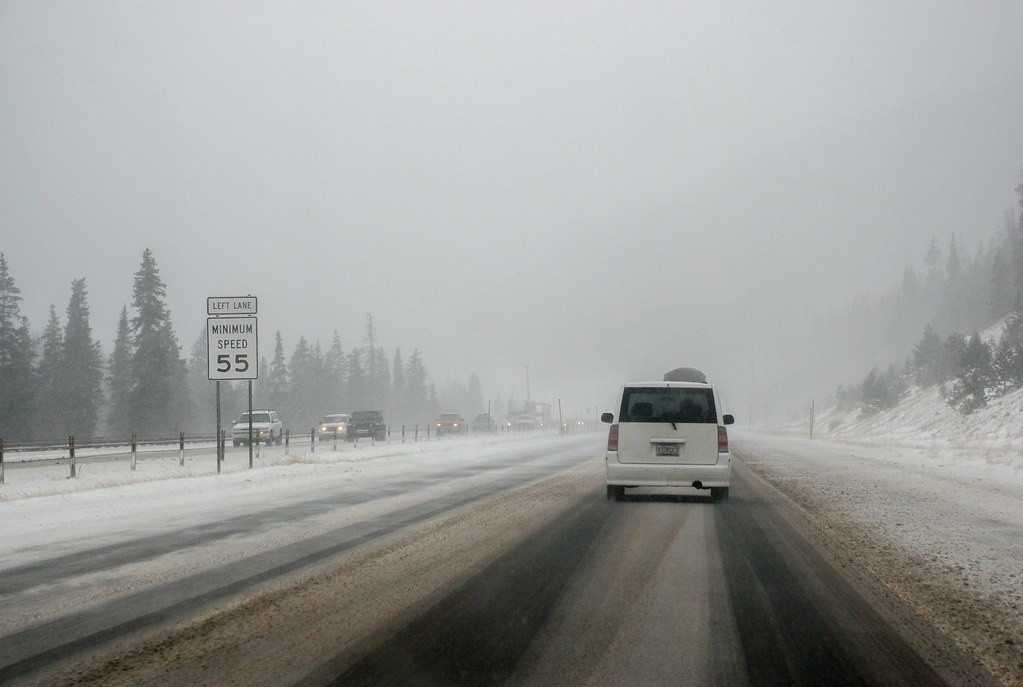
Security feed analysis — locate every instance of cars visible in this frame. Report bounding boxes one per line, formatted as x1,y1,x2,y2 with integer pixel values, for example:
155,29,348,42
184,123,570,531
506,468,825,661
601,381,734,502
232,409,282,448
434,400,551,436
319,411,386,442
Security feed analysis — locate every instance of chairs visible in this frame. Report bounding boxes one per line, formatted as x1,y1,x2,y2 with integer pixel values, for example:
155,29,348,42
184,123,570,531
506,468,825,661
630,403,654,417
680,405,702,418
